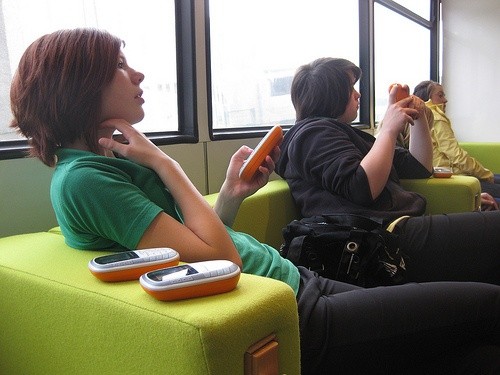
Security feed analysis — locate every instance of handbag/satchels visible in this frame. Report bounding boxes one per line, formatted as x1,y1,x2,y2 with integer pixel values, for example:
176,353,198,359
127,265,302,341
280,216,397,284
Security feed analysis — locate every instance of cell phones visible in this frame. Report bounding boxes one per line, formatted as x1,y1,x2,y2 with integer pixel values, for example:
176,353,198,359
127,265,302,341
388,84,409,115
89,247,181,282
138,260,241,302
239,125,282,182
433,166,453,178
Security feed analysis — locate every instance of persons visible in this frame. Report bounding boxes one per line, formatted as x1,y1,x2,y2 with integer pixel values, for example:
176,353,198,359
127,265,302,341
273,57,500,284
414,80,500,197
10,28,500,375
375,105,500,211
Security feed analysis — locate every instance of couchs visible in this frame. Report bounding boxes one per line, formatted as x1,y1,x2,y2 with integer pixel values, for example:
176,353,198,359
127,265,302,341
0,142,500,375
